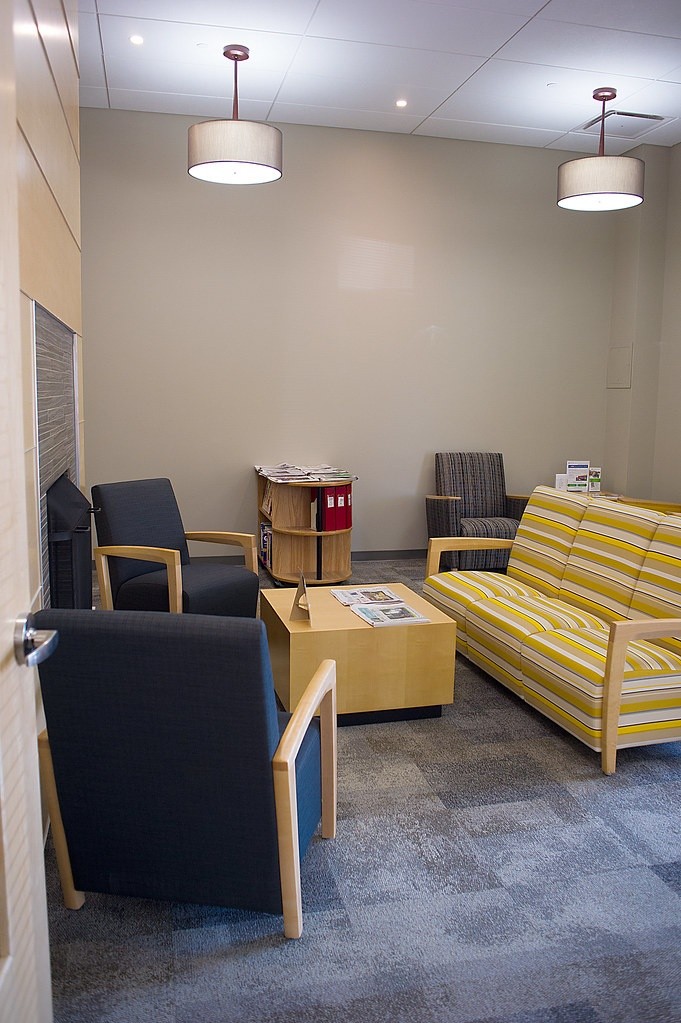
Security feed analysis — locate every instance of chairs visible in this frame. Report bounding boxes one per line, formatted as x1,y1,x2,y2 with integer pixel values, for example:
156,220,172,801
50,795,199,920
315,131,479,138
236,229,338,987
30,607,338,938
425,450,530,572
89,477,261,618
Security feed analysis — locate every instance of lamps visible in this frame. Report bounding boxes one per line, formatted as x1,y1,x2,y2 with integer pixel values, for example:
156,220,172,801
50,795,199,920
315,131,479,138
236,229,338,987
186,43,284,183
558,88,645,211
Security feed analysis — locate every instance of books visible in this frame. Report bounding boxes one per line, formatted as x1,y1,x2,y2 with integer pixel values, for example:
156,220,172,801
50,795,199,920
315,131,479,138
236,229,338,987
260,522,272,568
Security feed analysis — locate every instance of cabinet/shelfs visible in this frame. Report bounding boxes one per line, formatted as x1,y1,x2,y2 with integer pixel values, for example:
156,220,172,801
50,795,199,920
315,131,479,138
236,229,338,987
253,474,354,585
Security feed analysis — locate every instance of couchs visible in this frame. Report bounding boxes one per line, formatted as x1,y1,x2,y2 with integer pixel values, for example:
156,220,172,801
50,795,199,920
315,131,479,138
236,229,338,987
425,485,681,774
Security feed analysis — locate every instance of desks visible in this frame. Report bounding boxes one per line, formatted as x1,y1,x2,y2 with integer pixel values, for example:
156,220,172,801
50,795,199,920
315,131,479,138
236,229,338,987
258,583,458,726
